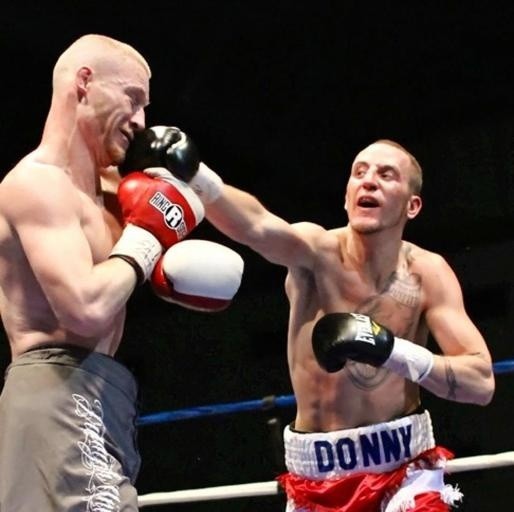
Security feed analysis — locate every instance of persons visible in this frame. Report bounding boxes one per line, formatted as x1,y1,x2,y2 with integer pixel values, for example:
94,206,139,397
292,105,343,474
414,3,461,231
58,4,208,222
0,33,248,512
119,124,496,511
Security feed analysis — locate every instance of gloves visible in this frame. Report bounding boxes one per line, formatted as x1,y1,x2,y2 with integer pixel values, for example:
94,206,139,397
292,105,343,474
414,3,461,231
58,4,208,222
116,166,205,250
118,124,200,183
312,313,394,373
151,238,245,314
